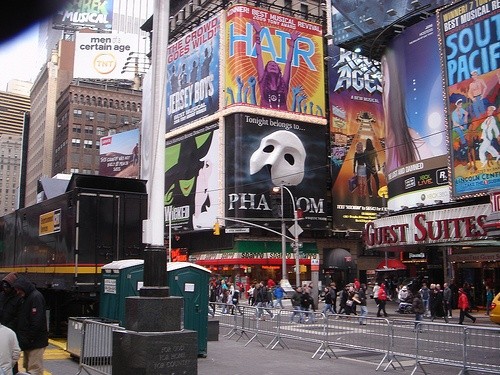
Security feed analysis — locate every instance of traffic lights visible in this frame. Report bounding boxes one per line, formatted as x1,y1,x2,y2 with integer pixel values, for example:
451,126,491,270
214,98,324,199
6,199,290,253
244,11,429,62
213,223,220,235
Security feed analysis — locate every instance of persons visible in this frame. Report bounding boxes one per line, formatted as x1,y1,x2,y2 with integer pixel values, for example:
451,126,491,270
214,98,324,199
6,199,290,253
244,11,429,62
411,282,477,332
0,322,21,375
0,271,23,374
486,284,494,315
12,276,49,374
170,38,214,126
209,275,287,322
224,18,324,118
289,278,402,326
132,143,138,167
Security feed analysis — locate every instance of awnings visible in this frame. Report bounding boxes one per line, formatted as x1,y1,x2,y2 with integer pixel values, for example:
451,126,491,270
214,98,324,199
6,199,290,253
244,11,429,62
375,260,407,270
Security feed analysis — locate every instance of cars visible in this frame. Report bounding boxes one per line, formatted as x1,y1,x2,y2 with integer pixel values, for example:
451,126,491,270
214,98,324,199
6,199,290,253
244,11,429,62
489,292,500,323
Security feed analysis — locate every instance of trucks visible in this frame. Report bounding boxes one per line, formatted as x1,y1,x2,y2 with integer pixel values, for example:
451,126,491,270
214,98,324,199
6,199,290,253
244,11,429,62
0,172,148,336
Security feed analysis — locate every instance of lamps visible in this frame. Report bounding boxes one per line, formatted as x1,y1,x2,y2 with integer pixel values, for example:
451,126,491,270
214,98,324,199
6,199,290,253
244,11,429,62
323,1,433,60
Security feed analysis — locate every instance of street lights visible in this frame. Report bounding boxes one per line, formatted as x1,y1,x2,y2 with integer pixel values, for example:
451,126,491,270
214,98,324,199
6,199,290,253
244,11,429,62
273,186,301,293
